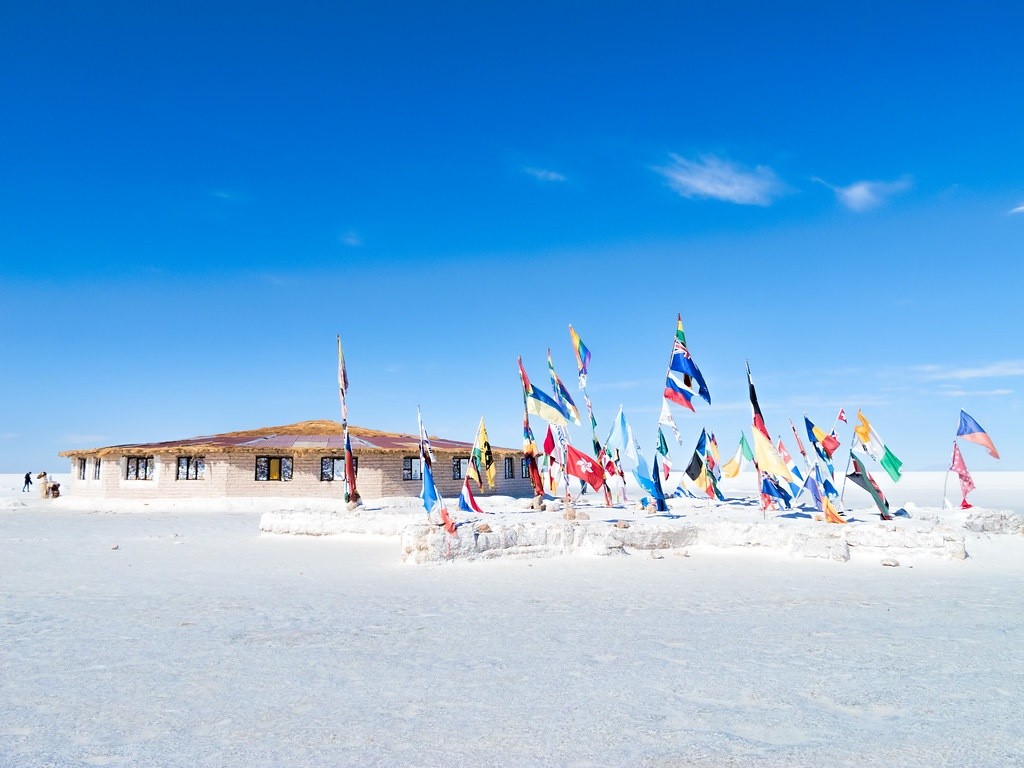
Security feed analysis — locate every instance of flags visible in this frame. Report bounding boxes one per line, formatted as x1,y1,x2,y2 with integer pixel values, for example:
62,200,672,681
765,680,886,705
337,312,1000,539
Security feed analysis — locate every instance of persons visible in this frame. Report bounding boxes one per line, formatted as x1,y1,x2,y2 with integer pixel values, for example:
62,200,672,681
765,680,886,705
22,471,32,492
